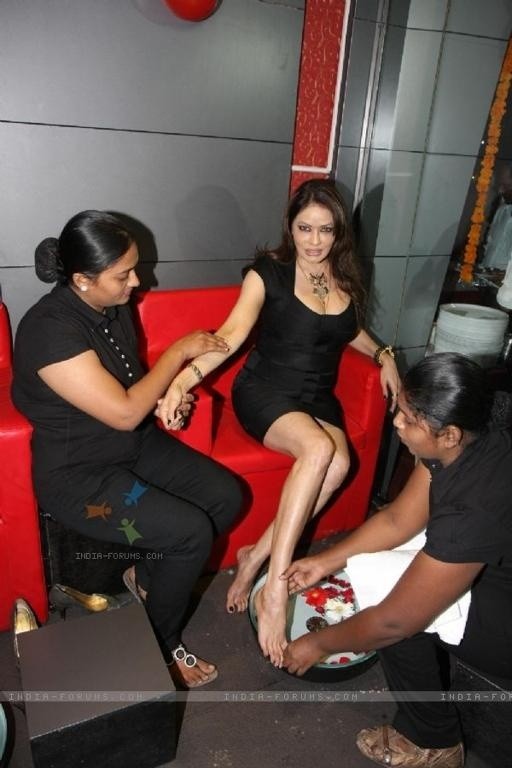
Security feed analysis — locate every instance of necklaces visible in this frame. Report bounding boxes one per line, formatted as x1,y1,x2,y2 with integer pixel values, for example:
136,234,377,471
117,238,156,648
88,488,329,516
295,256,330,308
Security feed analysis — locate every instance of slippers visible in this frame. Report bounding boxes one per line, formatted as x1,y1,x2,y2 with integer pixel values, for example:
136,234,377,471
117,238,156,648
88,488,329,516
123,566,146,605
164,643,219,689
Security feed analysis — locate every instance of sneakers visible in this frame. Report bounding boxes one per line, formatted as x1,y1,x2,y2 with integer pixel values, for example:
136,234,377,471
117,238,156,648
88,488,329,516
356,725,465,767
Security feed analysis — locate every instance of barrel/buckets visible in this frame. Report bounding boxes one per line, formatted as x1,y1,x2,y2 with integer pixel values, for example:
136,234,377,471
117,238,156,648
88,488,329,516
434,303,509,372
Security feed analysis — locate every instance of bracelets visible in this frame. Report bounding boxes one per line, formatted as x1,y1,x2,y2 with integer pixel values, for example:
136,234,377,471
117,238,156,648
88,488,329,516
183,360,205,385
373,344,397,368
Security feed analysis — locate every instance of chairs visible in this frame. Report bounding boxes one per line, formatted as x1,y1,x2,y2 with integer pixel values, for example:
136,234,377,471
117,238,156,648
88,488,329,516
1,286,385,634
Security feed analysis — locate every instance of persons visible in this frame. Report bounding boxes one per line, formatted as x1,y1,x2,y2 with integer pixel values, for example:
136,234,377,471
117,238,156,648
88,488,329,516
159,183,404,670
10,209,245,692
280,351,512,768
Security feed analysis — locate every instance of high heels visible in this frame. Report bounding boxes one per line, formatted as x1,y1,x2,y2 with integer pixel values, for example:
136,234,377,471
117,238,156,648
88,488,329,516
49,584,127,620
11,598,41,669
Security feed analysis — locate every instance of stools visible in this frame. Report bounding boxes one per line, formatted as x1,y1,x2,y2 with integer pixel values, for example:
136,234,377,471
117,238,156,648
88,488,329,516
451,649,511,768
16,603,186,767
